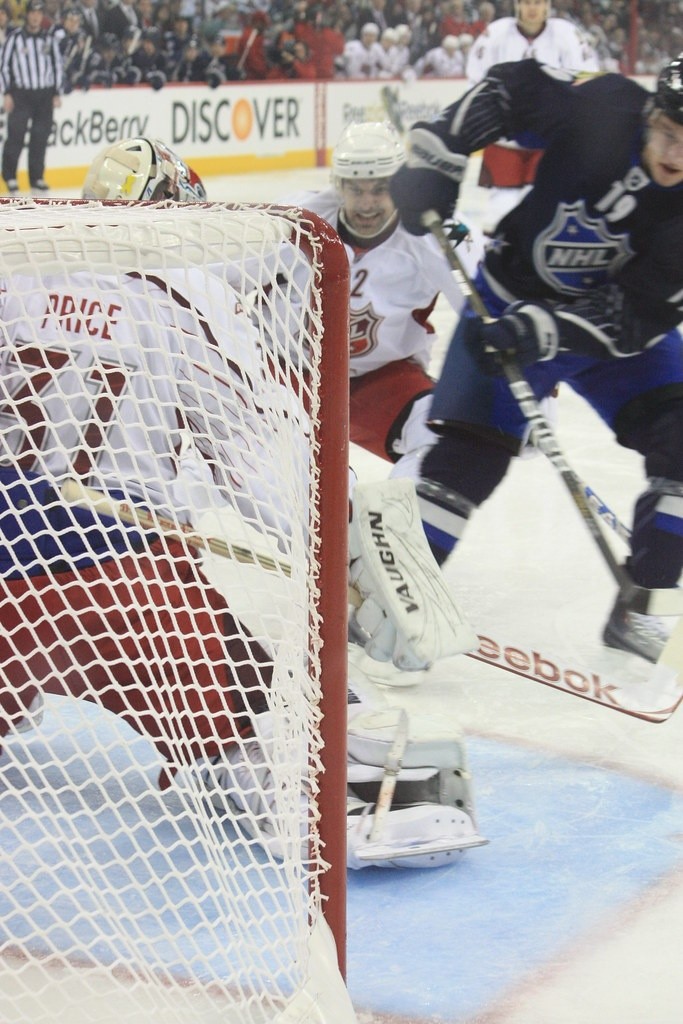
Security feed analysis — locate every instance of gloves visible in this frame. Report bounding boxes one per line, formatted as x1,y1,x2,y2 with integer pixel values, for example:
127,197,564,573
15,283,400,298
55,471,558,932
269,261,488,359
470,298,559,379
391,122,470,236
65,74,220,94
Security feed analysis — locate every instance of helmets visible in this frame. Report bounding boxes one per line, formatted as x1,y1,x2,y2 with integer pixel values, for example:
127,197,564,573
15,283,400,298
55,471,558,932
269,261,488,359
382,27,398,43
26,2,42,11
62,7,85,24
396,24,411,37
442,35,460,49
144,27,160,39
331,121,406,181
458,32,474,46
81,136,207,204
164,31,178,44
95,32,119,51
655,53,683,125
187,34,202,48
363,23,379,36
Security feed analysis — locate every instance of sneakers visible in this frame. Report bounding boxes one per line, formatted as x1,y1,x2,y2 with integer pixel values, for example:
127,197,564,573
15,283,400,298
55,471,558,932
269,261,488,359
320,798,490,870
602,613,670,664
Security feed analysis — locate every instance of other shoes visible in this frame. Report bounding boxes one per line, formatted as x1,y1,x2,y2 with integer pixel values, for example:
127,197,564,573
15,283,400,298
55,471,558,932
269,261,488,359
6,177,20,196
29,176,49,193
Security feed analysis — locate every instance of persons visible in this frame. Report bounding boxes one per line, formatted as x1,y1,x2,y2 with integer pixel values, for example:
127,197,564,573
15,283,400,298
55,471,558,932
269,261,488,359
204,119,552,484
350,47,683,686
464,0,600,273
0,138,488,871
0,0,683,94
0,0,63,192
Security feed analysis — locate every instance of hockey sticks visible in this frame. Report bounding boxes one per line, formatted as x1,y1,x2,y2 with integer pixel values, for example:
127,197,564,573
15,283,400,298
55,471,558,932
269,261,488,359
60,473,683,726
418,208,683,656
